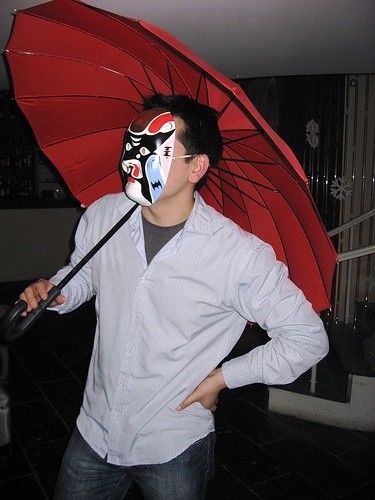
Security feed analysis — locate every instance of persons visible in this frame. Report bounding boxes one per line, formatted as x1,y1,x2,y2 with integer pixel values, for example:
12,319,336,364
16,95,330,499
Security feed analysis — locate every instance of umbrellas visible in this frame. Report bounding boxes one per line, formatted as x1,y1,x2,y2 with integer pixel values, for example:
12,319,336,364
3,0,339,314
